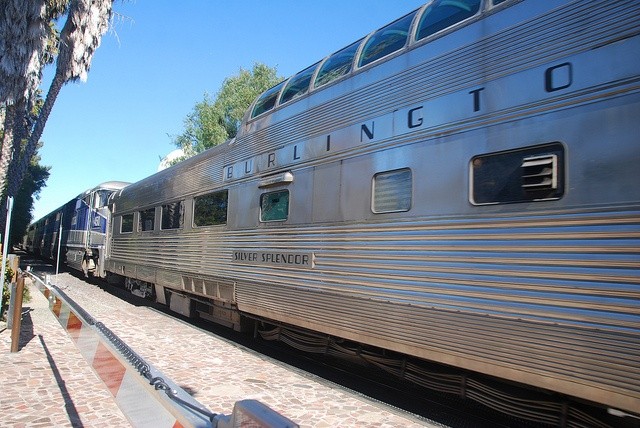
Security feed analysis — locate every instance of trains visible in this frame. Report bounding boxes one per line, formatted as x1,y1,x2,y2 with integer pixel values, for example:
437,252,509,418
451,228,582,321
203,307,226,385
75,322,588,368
22,1,640,427
